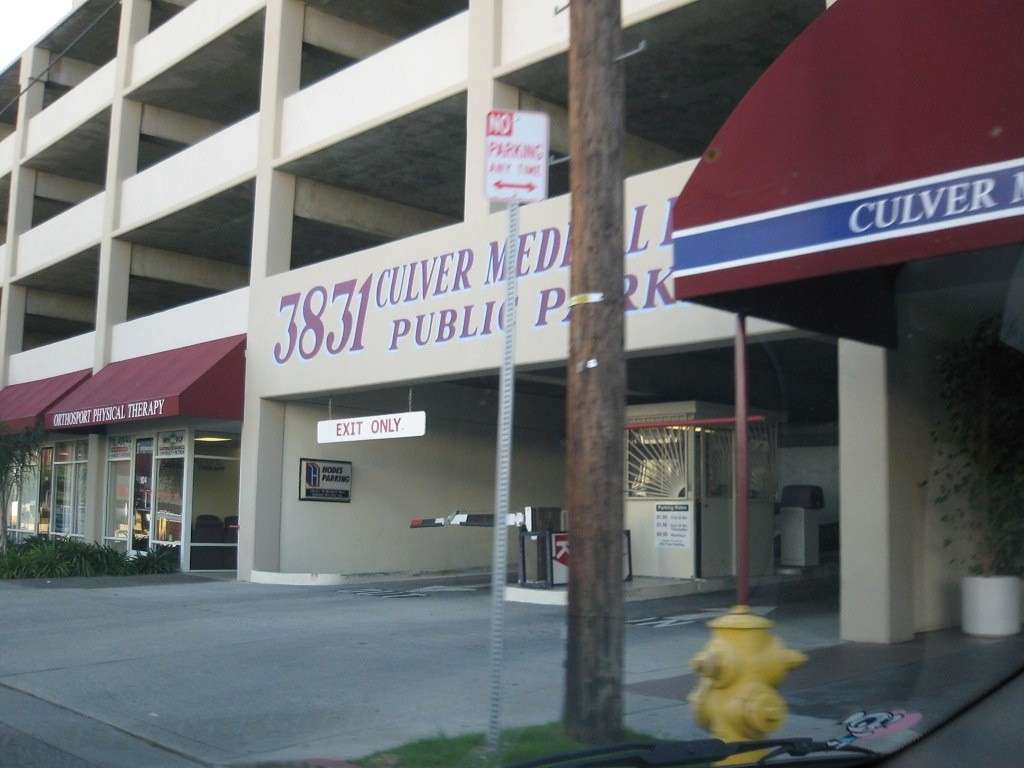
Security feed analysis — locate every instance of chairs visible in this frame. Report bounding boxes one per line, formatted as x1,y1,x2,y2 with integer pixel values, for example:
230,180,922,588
196,514,222,560
192,521,223,568
224,516,238,546
223,525,240,569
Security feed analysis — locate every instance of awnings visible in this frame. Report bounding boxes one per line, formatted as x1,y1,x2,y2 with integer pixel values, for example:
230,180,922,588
0,367,94,435
43,333,248,435
669,0,1024,351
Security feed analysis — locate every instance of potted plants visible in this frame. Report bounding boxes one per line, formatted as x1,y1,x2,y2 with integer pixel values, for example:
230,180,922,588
918,315,1024,639
